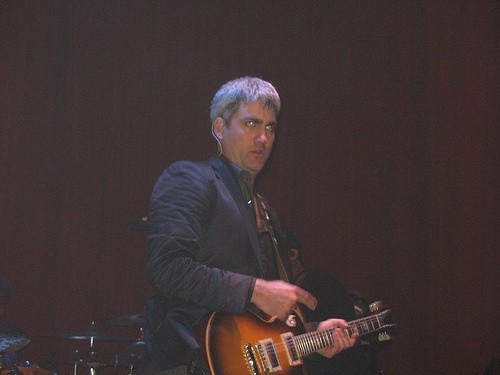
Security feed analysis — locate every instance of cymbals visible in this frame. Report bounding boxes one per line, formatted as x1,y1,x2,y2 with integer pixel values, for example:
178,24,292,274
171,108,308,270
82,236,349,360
62,356,111,367
64,331,129,342
103,315,149,328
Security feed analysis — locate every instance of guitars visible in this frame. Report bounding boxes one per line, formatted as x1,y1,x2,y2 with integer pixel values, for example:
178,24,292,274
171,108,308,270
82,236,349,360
195,300,395,374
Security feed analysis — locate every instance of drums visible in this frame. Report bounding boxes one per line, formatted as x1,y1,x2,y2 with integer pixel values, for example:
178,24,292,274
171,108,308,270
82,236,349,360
0,361,52,375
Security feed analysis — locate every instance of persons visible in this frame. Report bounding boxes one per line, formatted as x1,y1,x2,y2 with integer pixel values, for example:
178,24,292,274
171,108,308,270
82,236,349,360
140,76,361,373
